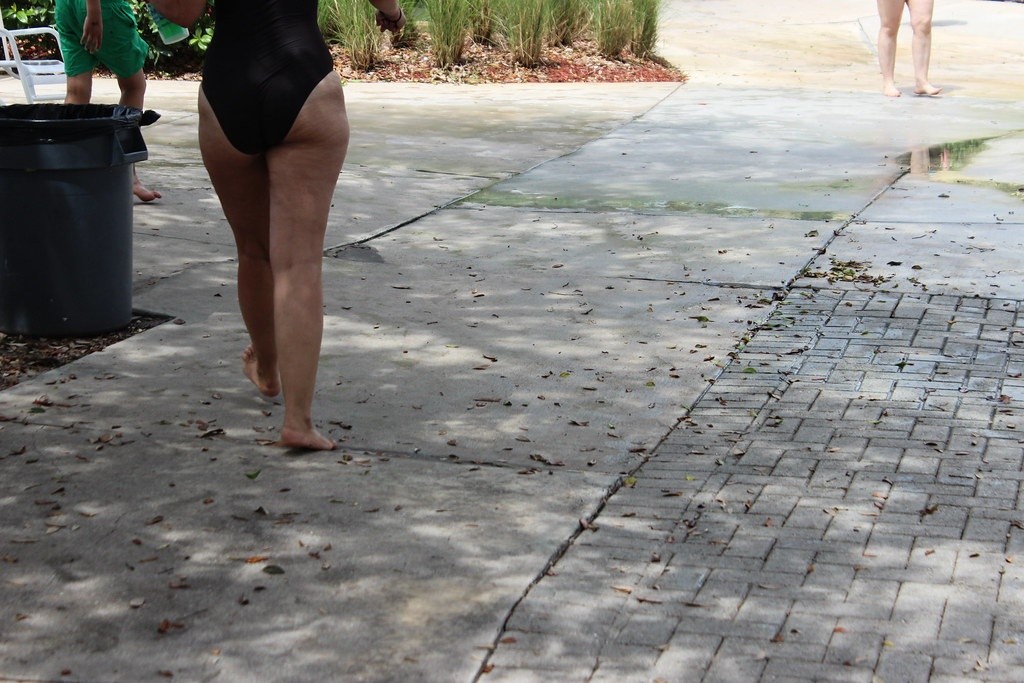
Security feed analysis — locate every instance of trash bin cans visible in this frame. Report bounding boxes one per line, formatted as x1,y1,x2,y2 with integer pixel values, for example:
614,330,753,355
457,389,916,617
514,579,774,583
0,102,161,338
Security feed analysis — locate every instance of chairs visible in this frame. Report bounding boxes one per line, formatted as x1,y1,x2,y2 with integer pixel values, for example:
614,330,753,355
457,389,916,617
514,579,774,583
1,5,67,104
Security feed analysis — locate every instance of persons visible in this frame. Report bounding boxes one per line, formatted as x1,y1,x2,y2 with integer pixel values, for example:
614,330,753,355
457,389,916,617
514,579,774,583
55,1,164,202
149,0,407,454
875,1,943,97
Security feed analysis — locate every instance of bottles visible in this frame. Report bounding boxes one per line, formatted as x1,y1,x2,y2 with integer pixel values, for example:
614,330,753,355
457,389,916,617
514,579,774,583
146,1,191,45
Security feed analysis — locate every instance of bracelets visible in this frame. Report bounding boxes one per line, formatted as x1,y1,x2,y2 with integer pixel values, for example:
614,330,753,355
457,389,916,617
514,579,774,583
378,8,402,29
